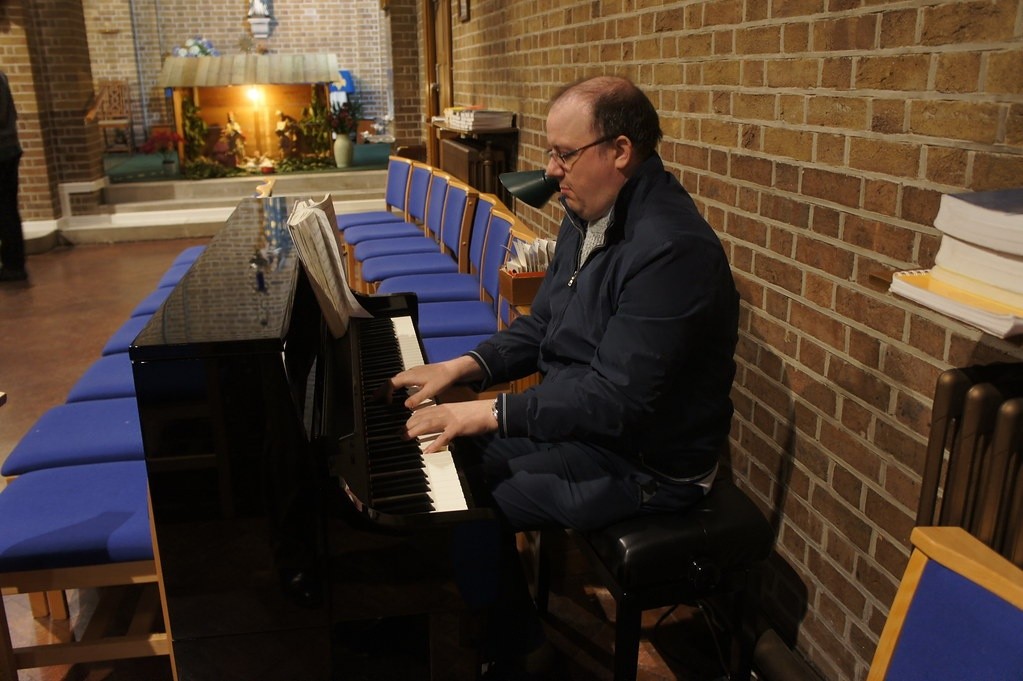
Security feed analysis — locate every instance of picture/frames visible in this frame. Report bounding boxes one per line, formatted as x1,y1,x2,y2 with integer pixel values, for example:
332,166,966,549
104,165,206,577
456,0,470,22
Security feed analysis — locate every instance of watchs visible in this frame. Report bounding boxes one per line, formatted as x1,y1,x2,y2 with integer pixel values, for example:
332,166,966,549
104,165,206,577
492,399,500,420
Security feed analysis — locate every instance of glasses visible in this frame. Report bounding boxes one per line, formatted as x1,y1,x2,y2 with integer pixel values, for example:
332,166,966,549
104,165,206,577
546,134,617,173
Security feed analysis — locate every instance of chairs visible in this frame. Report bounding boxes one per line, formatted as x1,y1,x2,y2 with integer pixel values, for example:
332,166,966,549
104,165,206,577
422,225,540,401
871,526,1023,681
418,209,522,336
344,161,433,288
360,180,479,292
375,193,509,300
336,155,412,247
353,169,450,293
0,245,209,681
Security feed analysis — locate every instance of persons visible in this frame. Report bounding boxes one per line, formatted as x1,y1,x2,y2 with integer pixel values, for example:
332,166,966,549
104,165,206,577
276,112,297,156
0,70,29,280
380,74,743,681
226,113,245,164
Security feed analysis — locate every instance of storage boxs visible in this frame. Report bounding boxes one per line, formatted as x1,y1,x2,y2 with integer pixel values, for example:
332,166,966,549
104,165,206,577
499,266,545,306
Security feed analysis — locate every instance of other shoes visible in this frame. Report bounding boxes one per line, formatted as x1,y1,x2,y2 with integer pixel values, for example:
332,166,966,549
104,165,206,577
478,592,545,681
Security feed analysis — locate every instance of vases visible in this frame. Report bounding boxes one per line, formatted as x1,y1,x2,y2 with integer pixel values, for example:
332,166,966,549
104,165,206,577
162,160,177,180
333,133,352,168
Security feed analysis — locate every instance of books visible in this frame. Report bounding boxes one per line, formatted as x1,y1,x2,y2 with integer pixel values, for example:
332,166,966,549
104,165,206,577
443,105,513,130
888,190,1023,338
288,195,369,337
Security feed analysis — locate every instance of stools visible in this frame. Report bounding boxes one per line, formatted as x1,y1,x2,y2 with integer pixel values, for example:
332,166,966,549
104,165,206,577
576,492,773,681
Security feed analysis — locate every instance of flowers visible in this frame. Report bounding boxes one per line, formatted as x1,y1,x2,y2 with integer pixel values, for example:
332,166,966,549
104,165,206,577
137,128,188,159
171,35,219,57
323,99,362,134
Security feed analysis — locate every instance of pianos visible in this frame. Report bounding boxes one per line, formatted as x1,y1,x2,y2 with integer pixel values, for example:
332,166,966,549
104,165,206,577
128,193,482,681
